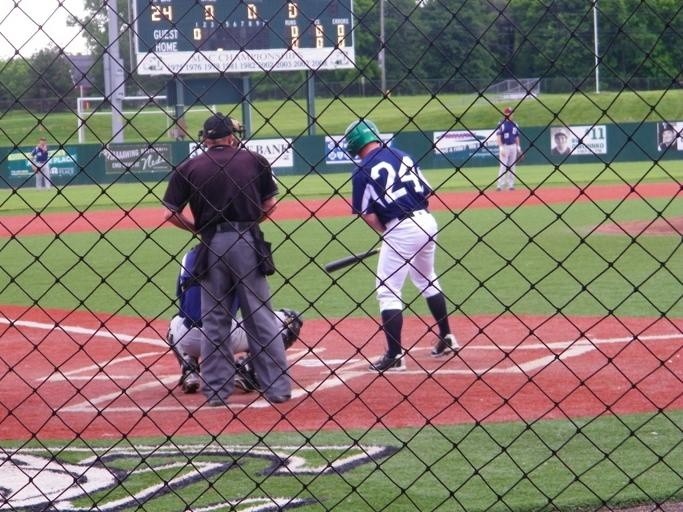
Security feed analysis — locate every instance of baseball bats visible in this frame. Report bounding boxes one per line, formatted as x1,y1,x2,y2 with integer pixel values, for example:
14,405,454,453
326,247,380,273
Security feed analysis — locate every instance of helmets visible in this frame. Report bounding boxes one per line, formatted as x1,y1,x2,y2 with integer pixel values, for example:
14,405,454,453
344,118,384,157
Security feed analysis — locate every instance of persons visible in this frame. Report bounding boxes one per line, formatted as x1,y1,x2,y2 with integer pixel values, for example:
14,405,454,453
345,118,458,373
657,123,679,152
30,136,52,190
166,236,303,394
496,107,525,191
551,128,570,156
163,111,292,407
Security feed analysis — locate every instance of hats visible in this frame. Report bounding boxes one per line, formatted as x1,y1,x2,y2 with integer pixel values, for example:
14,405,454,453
504,107,514,114
203,112,245,139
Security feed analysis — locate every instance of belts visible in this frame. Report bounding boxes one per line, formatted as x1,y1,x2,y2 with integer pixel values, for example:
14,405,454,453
211,221,250,234
384,209,432,229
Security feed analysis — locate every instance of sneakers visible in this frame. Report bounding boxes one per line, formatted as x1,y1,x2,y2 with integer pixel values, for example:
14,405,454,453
431,334,459,357
233,358,257,392
368,352,407,373
497,187,515,191
179,365,200,394
270,395,289,403
208,393,224,407
